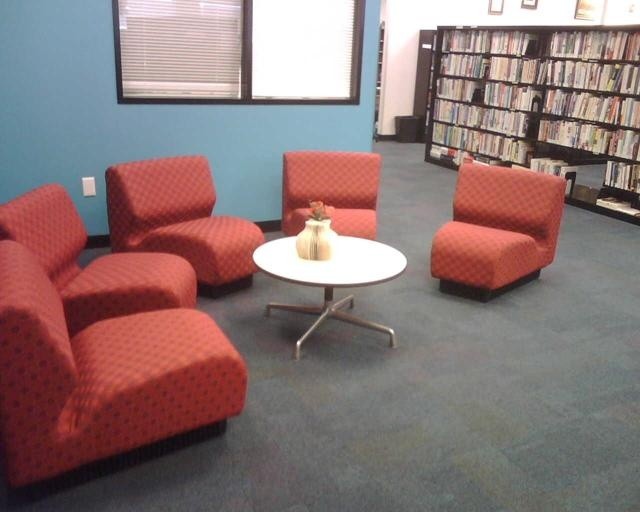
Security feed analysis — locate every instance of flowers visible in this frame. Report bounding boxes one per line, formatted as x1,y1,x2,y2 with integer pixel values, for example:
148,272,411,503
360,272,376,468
306,198,336,221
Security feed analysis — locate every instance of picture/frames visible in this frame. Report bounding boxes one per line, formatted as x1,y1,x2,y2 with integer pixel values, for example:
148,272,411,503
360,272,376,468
488,0,504,16
521,0,538,10
575,1,596,22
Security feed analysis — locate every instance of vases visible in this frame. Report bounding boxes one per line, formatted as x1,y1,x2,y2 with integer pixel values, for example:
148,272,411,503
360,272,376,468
295,218,344,262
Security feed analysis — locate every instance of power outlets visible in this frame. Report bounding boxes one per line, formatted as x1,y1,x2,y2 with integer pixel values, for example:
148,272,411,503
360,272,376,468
81,177,97,197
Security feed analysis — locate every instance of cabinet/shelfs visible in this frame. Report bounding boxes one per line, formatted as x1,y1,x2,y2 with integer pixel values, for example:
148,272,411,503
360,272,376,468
422,26,640,229
414,29,437,131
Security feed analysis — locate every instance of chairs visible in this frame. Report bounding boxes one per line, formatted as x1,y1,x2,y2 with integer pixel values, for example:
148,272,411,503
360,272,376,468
282,150,382,246
1,181,198,327
105,154,265,295
429,161,570,299
0,238,248,490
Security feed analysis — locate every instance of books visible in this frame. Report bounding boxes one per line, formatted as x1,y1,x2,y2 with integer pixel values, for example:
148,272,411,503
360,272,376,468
430,29,640,216
